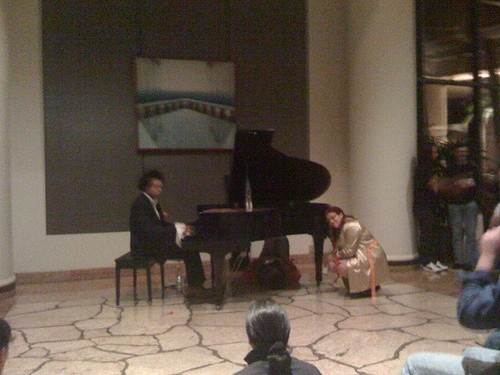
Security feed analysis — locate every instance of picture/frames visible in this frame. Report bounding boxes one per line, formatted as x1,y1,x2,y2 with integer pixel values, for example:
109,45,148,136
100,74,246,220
134,57,238,152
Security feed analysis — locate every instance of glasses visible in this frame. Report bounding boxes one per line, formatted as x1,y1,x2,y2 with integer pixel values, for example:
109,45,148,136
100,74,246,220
327,214,337,224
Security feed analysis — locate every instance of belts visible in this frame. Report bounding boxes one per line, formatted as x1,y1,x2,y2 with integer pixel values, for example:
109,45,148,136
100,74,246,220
447,199,472,205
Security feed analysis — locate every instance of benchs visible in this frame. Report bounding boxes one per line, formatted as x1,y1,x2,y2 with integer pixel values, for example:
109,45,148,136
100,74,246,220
115,251,166,302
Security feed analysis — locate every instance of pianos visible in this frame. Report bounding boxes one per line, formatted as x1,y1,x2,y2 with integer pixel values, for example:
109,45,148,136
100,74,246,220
181,129,331,304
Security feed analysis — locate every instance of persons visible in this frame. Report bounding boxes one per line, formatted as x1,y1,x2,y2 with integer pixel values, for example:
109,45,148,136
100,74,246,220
398,222,500,375
229,234,303,294
444,139,484,273
321,206,392,300
436,137,500,230
231,298,322,375
412,143,452,268
0,317,12,375
128,169,213,299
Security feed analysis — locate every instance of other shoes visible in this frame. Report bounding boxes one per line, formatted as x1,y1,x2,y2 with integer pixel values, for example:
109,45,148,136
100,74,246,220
185,285,212,302
350,285,381,299
435,260,448,271
452,264,463,269
462,263,472,270
423,262,441,273
